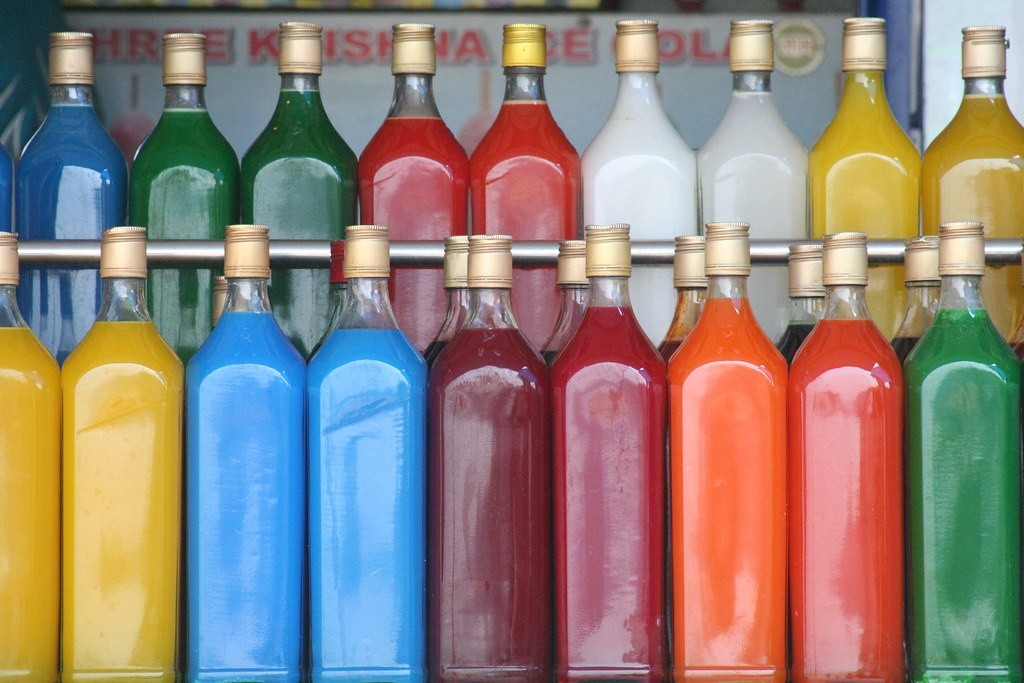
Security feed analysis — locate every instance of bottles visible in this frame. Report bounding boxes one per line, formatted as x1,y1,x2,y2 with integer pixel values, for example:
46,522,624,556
469,23,581,355
129,34,240,374
696,20,809,346
239,23,358,362
0,219,1023,683
920,28,1024,344
807,16,921,343
581,21,696,350
357,24,469,356
1,30,128,365
